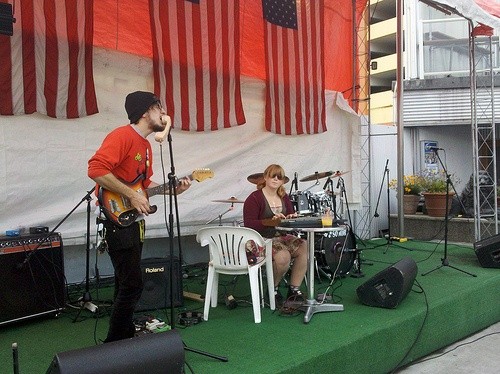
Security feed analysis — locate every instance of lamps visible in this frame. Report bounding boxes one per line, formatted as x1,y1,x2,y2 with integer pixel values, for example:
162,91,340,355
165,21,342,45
370,62,377,71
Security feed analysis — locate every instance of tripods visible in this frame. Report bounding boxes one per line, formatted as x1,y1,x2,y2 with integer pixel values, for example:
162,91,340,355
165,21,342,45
374,159,412,254
23,186,114,323
421,150,477,277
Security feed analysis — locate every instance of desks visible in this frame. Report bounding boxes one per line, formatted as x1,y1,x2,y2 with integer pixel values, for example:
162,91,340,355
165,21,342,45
275,224,349,321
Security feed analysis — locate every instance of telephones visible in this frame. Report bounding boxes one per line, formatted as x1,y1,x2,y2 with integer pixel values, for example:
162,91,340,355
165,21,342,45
155,114,172,142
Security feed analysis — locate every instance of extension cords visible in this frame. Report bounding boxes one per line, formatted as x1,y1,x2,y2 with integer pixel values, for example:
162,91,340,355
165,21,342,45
183,291,205,303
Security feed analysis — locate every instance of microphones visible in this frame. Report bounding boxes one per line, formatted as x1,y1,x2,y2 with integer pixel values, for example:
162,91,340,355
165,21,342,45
323,177,330,190
374,213,379,217
294,173,298,190
425,145,443,150
337,178,340,188
338,247,358,255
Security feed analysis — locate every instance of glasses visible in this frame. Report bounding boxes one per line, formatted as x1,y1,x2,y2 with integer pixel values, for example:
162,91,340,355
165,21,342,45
268,173,283,180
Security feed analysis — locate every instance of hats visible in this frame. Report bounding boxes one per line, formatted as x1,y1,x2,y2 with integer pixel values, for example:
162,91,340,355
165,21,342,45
125,91,159,124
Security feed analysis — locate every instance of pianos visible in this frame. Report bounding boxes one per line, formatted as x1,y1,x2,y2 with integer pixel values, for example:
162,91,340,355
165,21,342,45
279,215,337,227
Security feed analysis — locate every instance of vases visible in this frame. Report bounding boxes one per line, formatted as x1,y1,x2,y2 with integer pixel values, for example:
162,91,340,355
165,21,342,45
422,192,456,217
396,194,420,214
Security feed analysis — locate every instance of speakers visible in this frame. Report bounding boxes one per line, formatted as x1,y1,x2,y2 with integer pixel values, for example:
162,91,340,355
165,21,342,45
114,257,183,313
46,330,185,374
473,233,500,269
356,257,418,309
0,232,66,329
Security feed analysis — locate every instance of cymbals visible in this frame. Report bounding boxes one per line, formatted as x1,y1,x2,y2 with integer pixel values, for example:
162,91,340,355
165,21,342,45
300,171,335,183
247,172,289,184
212,199,245,203
330,170,351,178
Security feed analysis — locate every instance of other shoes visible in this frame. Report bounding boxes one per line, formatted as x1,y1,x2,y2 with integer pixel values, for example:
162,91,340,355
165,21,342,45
267,284,286,304
287,284,307,301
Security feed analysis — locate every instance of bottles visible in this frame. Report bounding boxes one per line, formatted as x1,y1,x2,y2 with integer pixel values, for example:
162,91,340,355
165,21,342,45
416,195,425,212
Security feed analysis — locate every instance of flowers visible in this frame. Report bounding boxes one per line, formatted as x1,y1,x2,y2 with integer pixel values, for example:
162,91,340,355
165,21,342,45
388,169,450,194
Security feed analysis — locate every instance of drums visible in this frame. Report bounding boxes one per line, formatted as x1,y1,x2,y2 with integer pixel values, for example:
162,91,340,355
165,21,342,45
309,192,332,213
314,223,357,277
287,190,311,215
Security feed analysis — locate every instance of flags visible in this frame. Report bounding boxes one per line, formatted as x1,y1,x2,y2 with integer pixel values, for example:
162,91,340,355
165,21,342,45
0,0,327,136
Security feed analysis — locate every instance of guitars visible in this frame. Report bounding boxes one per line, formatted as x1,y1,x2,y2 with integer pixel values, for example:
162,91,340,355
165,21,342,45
99,167,214,227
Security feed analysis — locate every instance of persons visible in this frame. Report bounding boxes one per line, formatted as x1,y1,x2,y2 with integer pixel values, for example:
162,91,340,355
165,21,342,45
243,164,308,307
88,91,192,343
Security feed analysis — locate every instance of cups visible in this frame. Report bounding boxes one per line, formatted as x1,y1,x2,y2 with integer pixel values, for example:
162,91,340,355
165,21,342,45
322,214,333,227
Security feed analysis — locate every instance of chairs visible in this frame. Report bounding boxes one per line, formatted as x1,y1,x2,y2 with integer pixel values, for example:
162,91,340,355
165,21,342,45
196,226,275,323
